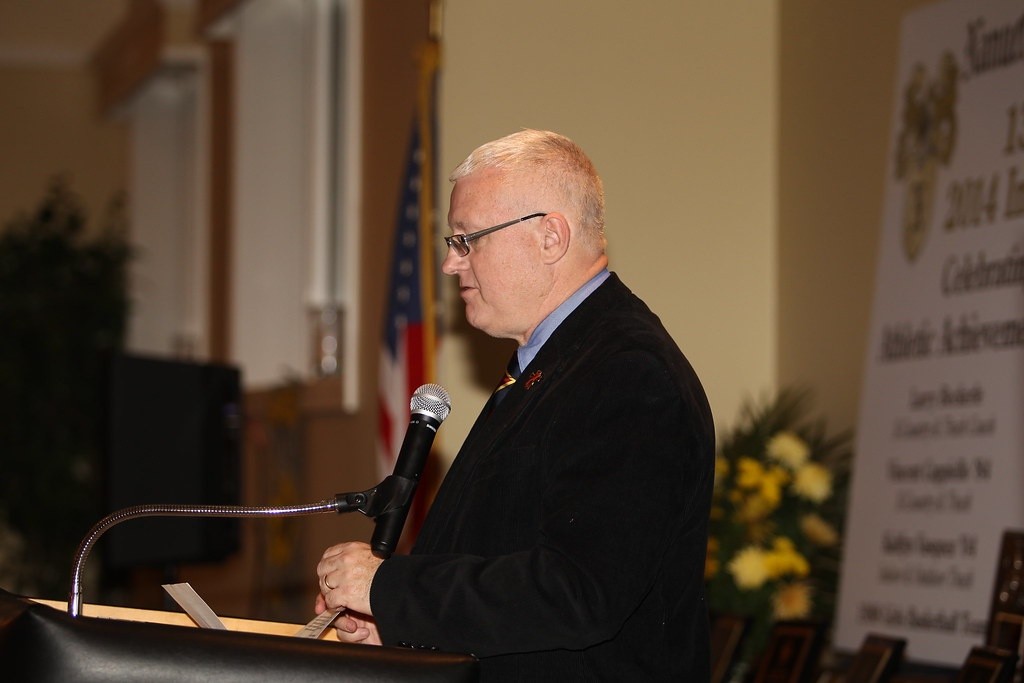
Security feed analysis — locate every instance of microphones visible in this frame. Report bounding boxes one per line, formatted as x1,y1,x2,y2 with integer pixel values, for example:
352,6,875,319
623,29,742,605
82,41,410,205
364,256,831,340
370,382,451,560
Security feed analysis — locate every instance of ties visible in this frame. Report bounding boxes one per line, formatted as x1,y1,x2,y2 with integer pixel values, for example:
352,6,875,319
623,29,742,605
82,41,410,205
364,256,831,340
482,351,521,425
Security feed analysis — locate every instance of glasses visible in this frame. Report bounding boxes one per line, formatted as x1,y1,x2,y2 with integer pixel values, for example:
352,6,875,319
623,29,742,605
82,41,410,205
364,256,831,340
443,213,546,258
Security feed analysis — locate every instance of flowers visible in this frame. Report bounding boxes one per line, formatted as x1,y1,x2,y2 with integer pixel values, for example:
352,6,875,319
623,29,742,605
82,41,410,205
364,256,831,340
702,382,855,651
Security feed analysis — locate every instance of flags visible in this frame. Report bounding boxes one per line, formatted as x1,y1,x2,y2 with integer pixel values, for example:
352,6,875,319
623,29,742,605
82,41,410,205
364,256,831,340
374,75,442,557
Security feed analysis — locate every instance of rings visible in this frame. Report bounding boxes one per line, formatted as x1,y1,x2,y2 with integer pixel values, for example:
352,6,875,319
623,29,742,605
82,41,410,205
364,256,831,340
325,575,335,590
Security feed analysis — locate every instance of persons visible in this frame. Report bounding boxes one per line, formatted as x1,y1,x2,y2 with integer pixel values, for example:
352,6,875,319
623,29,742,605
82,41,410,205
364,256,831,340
314,125,715,683
